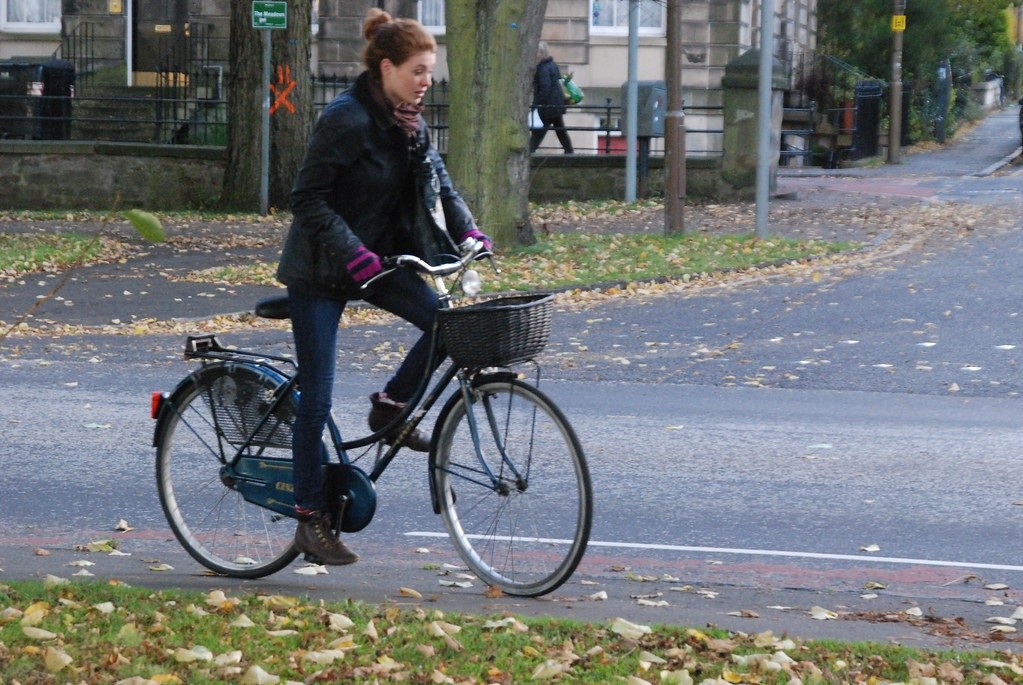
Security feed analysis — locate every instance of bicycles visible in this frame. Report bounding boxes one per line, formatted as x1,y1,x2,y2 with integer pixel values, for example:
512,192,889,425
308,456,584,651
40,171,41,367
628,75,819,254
147,235,595,602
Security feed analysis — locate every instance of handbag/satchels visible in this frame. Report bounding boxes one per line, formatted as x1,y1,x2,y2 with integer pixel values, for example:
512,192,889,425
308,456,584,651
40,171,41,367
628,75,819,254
558,73,584,104
527,108,544,127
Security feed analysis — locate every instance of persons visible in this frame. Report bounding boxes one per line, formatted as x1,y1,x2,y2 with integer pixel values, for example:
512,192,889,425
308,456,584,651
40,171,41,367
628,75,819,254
271,7,493,566
529,42,574,154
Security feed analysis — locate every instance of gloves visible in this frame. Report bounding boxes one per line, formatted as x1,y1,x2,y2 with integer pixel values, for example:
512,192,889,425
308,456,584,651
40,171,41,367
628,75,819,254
345,245,382,284
463,229,492,260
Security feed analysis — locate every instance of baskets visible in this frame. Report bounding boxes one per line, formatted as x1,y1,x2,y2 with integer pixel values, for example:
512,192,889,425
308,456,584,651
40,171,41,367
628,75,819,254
438,293,554,366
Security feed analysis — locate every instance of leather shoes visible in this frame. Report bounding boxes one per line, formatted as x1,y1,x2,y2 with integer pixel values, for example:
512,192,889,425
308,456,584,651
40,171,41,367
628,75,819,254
295,510,359,565
369,392,431,452
